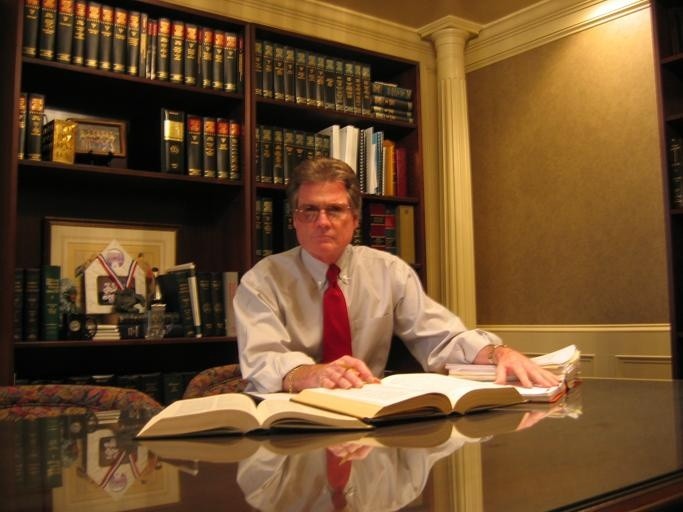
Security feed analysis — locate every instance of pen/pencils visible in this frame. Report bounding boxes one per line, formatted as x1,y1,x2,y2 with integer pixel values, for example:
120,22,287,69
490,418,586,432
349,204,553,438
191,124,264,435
335,360,353,369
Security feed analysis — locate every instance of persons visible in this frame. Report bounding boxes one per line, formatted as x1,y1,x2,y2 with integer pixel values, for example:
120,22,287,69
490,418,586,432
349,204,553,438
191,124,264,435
235,402,571,511
76,128,118,153
65,285,79,313
228,157,564,402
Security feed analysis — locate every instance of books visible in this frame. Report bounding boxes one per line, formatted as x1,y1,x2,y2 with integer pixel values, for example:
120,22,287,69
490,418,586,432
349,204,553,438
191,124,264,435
155,262,226,339
288,371,527,425
349,202,414,266
0,408,66,496
256,198,299,265
131,390,374,442
89,322,186,340
255,124,409,197
160,109,240,179
370,409,525,452
22,1,243,92
443,342,580,406
13,265,61,342
136,427,370,463
253,38,412,123
18,91,45,160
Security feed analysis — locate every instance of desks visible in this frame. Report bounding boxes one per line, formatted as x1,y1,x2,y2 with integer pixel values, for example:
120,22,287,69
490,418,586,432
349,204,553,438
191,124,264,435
1,380,681,511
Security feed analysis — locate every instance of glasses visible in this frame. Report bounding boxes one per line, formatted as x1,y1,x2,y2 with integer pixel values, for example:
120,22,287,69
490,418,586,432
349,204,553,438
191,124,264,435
289,205,355,222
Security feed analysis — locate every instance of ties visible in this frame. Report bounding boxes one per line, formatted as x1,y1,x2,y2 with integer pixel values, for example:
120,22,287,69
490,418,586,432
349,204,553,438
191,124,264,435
323,264,353,365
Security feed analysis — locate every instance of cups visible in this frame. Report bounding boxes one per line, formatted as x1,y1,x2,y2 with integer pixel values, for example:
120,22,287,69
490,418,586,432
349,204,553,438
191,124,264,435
61,311,97,341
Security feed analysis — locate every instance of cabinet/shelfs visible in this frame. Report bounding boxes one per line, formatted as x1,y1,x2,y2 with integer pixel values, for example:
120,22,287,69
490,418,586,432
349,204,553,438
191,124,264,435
0,0,428,385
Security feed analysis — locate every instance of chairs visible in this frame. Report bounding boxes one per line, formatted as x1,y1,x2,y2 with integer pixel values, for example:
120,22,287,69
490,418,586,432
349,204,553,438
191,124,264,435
1,384,164,421
182,364,249,398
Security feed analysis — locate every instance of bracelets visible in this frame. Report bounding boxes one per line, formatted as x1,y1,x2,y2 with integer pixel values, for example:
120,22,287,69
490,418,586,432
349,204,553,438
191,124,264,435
488,343,510,365
